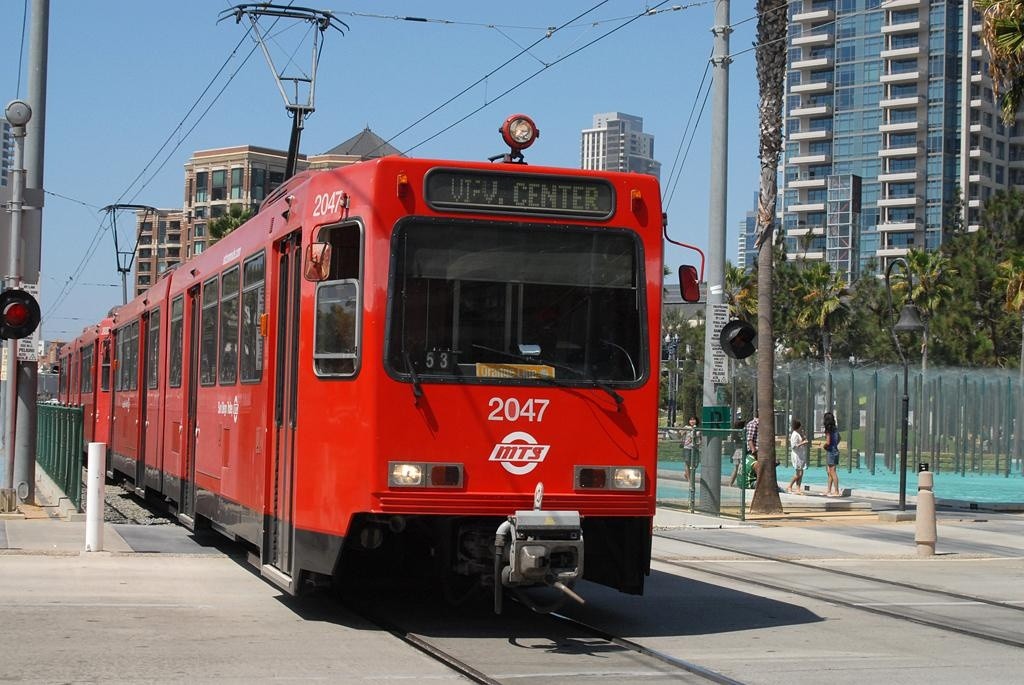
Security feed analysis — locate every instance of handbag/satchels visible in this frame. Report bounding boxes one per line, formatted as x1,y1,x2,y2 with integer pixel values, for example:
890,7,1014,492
730,454,740,463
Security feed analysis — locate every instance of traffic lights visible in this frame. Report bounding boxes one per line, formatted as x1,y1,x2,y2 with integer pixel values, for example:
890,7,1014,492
0,289,41,339
720,319,758,360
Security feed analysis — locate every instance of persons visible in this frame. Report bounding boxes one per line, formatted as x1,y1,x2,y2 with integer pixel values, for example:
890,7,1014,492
729,408,759,489
681,415,702,483
786,419,808,496
822,413,841,496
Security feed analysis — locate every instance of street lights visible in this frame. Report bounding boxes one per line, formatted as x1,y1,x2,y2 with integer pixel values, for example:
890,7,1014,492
664,331,680,431
848,354,856,474
885,256,925,512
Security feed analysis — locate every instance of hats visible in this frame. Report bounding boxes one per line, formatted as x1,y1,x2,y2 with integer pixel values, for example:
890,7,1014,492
733,420,745,430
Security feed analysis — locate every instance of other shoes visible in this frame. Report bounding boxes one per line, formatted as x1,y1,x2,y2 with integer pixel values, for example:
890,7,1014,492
786,487,792,494
796,491,804,495
819,492,840,497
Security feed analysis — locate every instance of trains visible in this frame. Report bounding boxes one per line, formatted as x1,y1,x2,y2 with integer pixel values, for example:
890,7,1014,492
57,0,706,624
